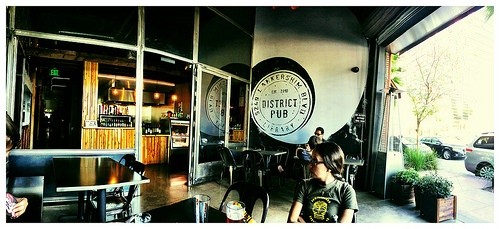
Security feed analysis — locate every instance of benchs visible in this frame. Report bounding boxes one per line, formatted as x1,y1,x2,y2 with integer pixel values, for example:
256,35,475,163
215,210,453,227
6,175,46,223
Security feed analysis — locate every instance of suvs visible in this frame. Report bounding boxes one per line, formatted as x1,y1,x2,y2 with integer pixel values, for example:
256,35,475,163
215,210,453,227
464,132,494,179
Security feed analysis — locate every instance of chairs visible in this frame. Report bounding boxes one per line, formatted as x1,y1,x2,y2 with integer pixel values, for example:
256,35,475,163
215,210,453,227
86,154,147,223
217,144,289,186
219,182,269,223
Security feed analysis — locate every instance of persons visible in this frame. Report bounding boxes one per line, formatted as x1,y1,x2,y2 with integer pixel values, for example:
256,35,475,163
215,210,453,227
6,112,28,223
286,127,329,201
287,142,354,223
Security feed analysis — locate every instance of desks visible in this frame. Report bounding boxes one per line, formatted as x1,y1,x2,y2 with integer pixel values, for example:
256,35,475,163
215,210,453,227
105,198,227,222
52,156,150,223
231,150,287,163
344,159,365,182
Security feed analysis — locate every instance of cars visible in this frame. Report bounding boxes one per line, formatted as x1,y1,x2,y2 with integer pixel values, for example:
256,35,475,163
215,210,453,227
420,135,467,160
392,135,431,154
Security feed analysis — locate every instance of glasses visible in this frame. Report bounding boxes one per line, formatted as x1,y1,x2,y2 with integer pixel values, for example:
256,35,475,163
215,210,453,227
315,132,322,136
309,158,324,166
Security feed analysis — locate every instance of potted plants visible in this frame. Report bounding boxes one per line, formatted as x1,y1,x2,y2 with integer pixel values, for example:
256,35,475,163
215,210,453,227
389,168,457,223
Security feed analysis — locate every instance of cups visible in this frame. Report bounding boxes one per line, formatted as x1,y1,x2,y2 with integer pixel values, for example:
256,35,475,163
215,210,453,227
225,200,247,223
6,192,19,218
193,194,211,223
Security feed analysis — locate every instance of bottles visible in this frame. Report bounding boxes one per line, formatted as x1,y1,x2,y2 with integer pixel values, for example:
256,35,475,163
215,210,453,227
99,102,135,127
162,111,189,119
142,122,161,135
